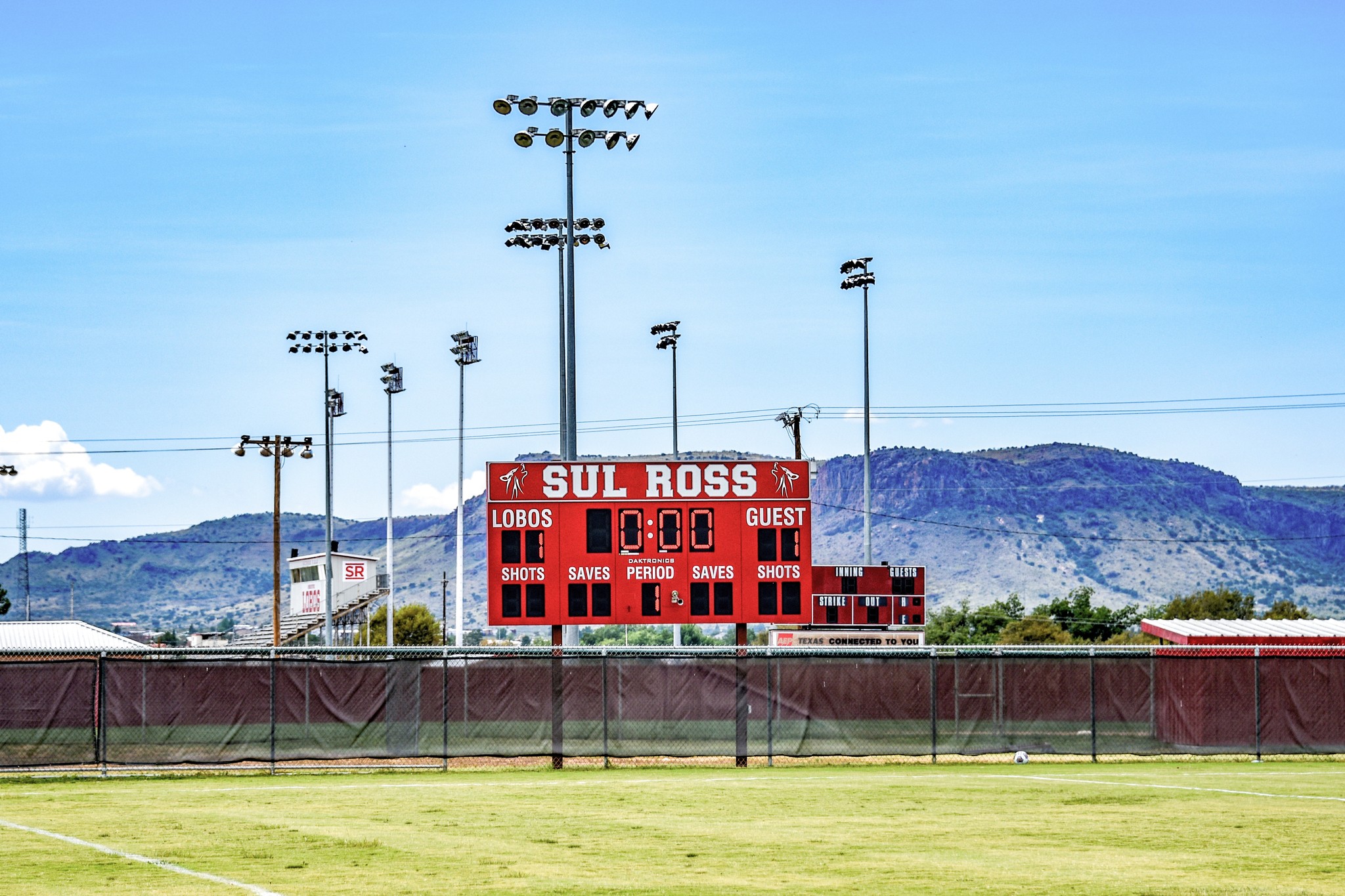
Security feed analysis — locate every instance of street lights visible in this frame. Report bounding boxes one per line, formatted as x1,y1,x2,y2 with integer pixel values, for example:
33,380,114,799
381,362,408,653
501,218,612,466
286,327,371,648
649,320,681,461
231,434,315,645
451,331,484,652
492,92,658,467
839,256,876,565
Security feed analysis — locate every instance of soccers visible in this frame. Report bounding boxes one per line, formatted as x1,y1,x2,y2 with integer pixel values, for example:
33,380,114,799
1013,751,1028,764
